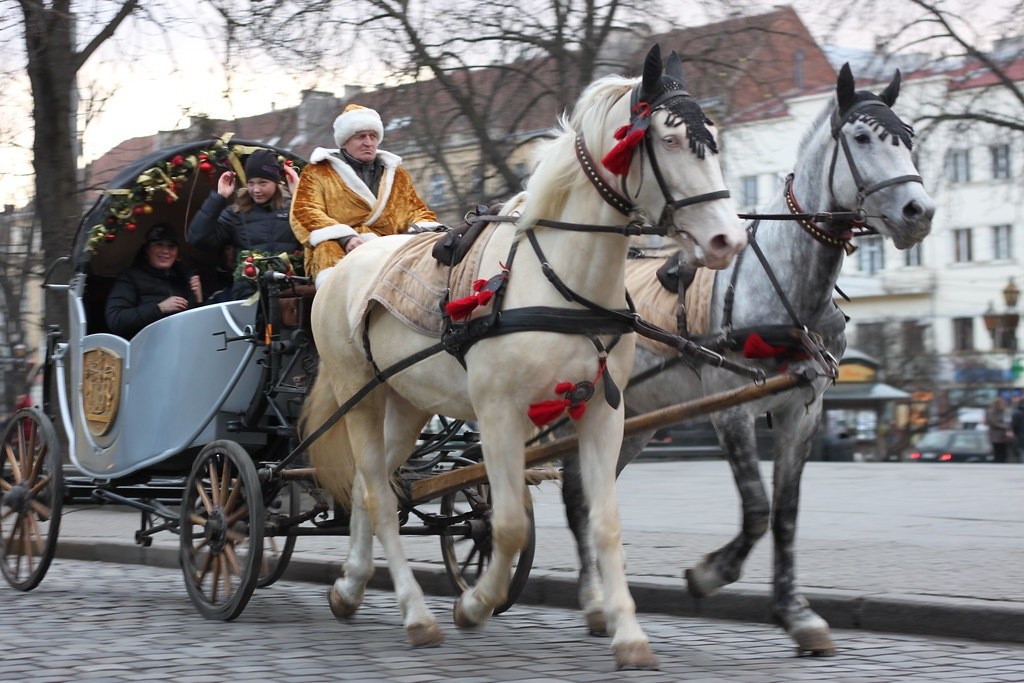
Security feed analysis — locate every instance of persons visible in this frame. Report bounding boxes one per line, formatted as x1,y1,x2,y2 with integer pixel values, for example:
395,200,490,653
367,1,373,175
289,103,441,291
11,394,40,448
187,149,303,432
1012,397,1024,461
988,398,1011,462
106,223,203,338
1002,396,1020,438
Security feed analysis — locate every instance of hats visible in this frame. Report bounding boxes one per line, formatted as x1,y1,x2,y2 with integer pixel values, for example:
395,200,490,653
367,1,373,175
145,223,180,247
245,150,282,185
333,103,385,149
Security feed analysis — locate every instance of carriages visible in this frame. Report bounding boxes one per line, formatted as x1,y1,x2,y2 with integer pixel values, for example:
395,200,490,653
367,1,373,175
1,42,939,675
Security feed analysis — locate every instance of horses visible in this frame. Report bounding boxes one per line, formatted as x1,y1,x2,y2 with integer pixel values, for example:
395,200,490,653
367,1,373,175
525,61,938,657
296,41,749,671
